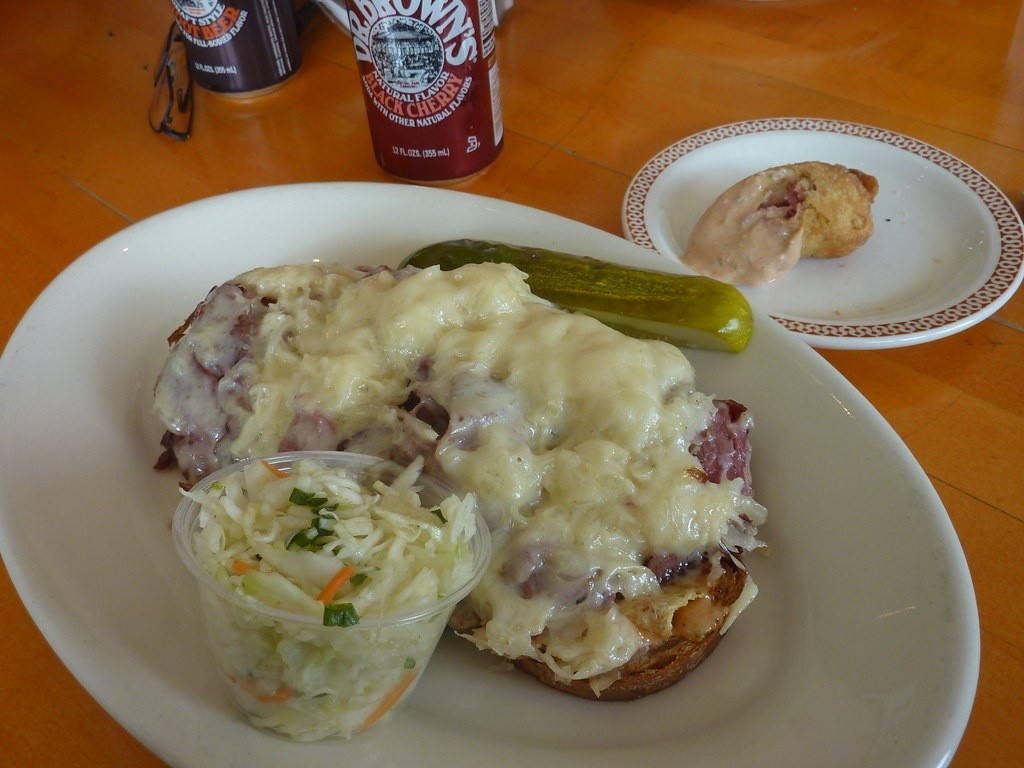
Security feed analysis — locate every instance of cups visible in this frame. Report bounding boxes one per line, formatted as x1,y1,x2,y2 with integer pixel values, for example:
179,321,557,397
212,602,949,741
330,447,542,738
171,450,492,743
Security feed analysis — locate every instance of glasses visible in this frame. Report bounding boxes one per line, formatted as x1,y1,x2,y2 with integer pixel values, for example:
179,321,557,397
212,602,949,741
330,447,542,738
148,21,196,138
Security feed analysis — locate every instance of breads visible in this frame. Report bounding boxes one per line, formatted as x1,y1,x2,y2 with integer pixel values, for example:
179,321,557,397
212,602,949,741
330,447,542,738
505,558,750,699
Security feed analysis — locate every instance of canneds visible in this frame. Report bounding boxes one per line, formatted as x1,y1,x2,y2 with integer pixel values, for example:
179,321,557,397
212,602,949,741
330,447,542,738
346,0,505,187
170,0,303,100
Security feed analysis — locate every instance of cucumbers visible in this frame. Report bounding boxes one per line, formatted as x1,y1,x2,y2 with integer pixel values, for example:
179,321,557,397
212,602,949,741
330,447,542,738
397,237,752,354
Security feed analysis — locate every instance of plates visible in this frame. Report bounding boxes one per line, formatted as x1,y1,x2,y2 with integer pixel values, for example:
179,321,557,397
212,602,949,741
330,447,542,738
0,181,982,768
621,117,1024,349
